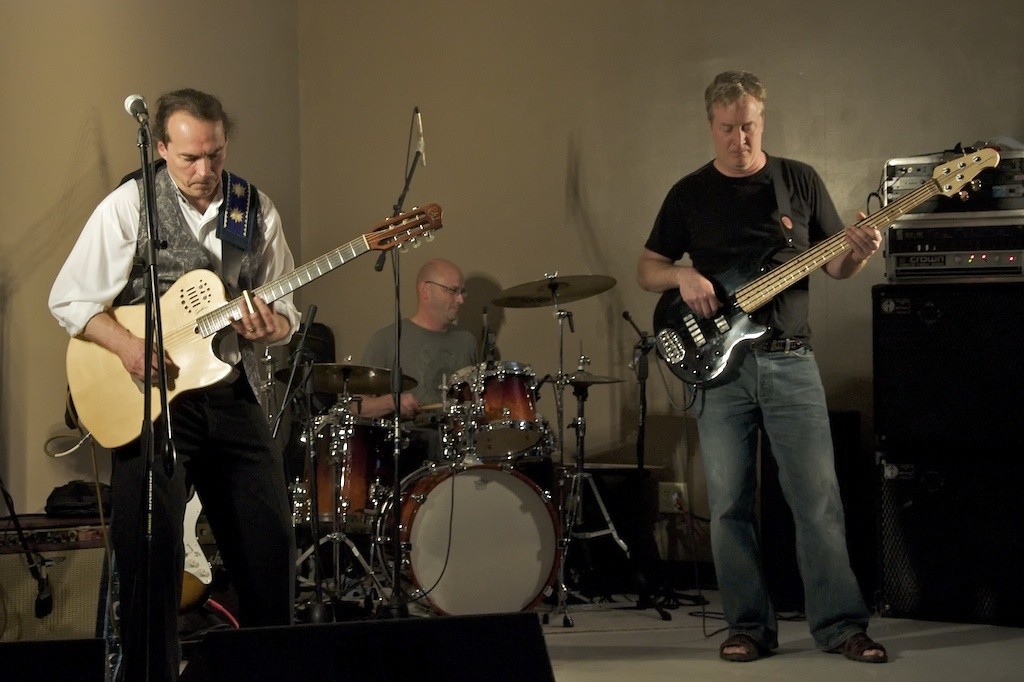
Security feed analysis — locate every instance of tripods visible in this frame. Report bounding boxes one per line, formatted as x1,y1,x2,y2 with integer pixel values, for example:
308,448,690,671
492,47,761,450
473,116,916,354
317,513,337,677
561,383,649,600
293,373,389,603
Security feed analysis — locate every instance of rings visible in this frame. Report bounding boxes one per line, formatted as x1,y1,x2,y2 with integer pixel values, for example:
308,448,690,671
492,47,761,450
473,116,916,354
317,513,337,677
247,327,255,335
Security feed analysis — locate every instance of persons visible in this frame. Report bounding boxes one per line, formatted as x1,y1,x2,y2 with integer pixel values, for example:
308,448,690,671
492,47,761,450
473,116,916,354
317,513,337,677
48,87,302,682
635,71,889,664
349,257,483,478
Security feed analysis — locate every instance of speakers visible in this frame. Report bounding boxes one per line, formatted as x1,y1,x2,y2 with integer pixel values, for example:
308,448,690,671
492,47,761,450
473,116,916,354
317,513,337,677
175,612,555,682
0,515,124,658
870,281,1024,630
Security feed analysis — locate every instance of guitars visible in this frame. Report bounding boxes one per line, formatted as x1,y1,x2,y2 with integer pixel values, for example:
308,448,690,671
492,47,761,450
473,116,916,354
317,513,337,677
651,140,1003,389
65,201,445,452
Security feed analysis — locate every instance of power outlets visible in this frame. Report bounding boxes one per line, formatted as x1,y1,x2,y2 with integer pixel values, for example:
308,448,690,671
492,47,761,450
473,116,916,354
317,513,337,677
659,482,688,513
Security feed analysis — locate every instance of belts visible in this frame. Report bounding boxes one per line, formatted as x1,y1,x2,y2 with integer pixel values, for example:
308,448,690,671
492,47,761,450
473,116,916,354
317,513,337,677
739,339,802,352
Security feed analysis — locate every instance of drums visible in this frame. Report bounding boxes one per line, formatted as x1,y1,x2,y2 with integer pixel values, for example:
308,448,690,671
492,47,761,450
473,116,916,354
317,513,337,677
443,360,545,461
298,412,425,534
375,461,564,617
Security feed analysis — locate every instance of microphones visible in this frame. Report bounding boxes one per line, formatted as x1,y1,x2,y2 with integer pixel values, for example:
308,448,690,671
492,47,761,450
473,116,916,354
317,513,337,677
480,306,494,342
124,94,149,126
415,106,426,167
33,558,52,618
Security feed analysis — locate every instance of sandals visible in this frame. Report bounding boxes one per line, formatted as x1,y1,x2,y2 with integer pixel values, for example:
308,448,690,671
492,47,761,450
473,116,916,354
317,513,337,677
832,632,888,662
720,633,779,662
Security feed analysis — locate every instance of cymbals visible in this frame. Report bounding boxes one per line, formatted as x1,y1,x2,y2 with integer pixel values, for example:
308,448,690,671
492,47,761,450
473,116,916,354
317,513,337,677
540,370,627,384
269,362,420,397
490,274,618,309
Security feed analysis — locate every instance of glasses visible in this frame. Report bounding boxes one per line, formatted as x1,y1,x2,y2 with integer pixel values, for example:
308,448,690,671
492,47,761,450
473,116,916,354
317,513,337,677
423,280,467,297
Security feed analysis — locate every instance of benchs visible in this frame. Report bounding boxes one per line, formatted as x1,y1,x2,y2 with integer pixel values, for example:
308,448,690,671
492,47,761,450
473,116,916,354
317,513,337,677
0,511,217,555
